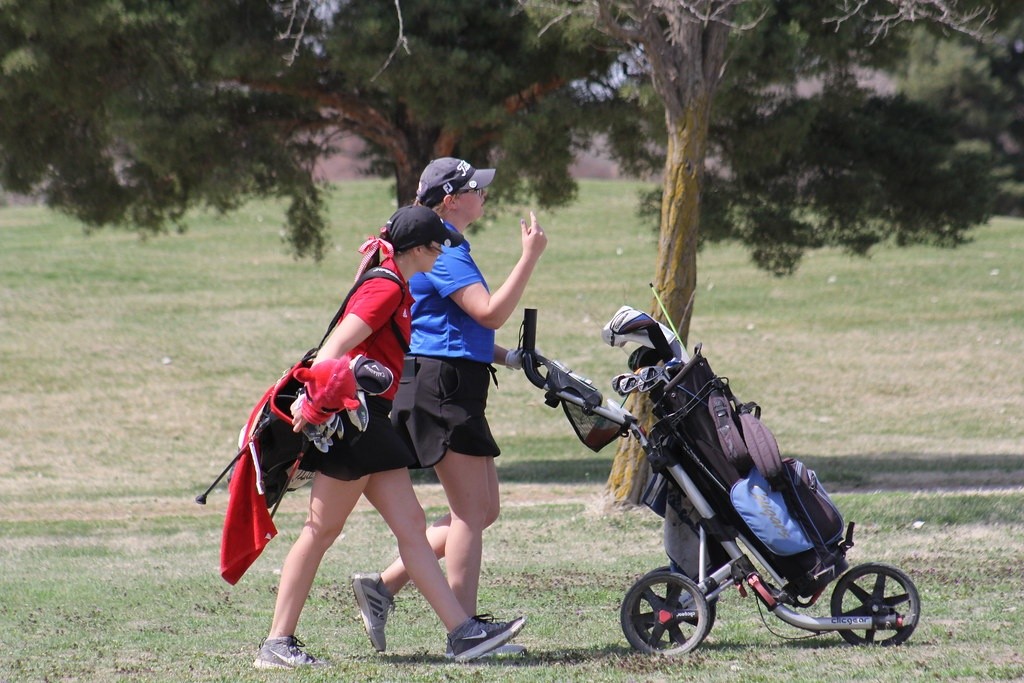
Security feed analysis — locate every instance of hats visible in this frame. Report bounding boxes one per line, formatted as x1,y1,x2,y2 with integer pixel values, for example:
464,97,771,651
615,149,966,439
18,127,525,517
383,206,464,251
416,158,496,207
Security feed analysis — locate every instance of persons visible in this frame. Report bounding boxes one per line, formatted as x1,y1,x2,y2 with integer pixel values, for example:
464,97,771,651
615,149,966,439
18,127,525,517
258,204,529,663
348,157,550,661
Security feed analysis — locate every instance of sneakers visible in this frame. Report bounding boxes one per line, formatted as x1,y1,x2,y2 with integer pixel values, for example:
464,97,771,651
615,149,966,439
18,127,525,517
445,633,526,657
353,572,391,652
447,616,526,663
253,638,329,670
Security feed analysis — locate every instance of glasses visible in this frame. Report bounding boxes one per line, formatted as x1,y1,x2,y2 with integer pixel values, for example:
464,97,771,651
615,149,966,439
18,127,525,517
459,188,484,196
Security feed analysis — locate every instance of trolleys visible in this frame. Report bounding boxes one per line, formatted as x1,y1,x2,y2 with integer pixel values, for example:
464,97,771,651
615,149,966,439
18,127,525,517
518,307,921,654
